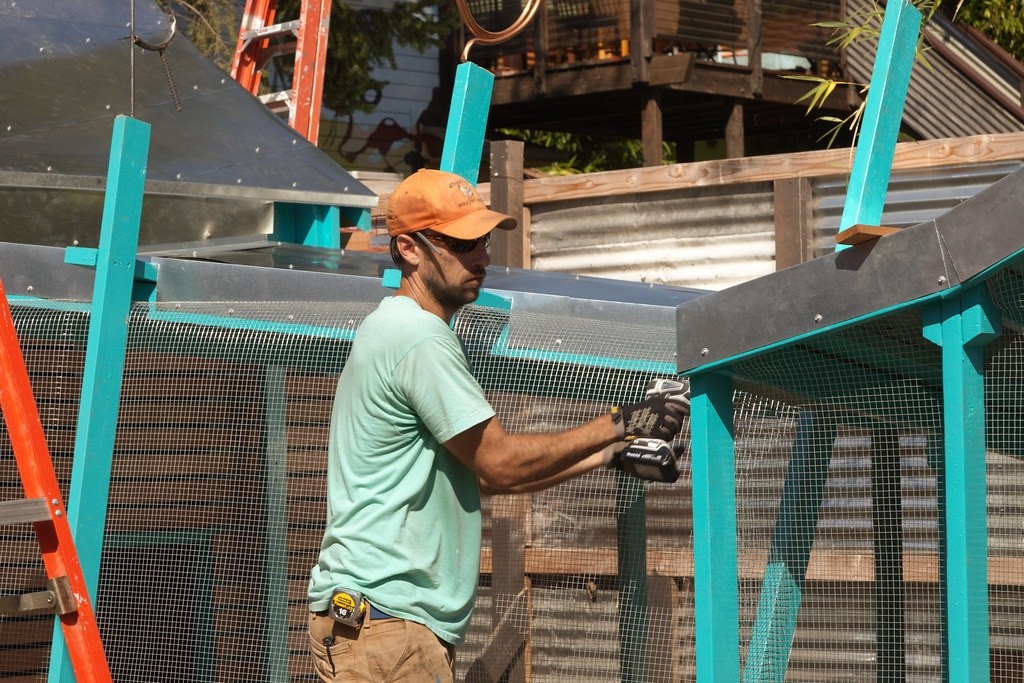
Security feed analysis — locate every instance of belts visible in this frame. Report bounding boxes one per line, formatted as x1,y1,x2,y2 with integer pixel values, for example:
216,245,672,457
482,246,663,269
370,604,393,619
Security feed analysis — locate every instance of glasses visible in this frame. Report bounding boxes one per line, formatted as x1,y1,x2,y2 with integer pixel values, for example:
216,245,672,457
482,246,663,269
423,230,493,254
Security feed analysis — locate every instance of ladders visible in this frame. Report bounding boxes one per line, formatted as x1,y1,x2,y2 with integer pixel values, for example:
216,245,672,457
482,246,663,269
0,276,114,683
229,0,332,147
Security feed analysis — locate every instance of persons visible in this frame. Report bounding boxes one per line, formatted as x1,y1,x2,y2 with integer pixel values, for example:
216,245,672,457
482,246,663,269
306,165,692,683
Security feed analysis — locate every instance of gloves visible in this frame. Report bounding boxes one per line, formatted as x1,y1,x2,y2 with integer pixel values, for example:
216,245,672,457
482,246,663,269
605,391,691,483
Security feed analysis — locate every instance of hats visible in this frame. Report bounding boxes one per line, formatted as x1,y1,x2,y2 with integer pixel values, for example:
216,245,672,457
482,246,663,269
386,167,518,241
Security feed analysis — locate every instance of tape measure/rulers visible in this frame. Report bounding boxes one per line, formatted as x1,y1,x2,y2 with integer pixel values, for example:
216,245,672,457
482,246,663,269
328,587,366,630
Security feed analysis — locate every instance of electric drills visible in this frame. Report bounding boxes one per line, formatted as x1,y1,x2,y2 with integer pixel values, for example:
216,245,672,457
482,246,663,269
620,377,690,483
